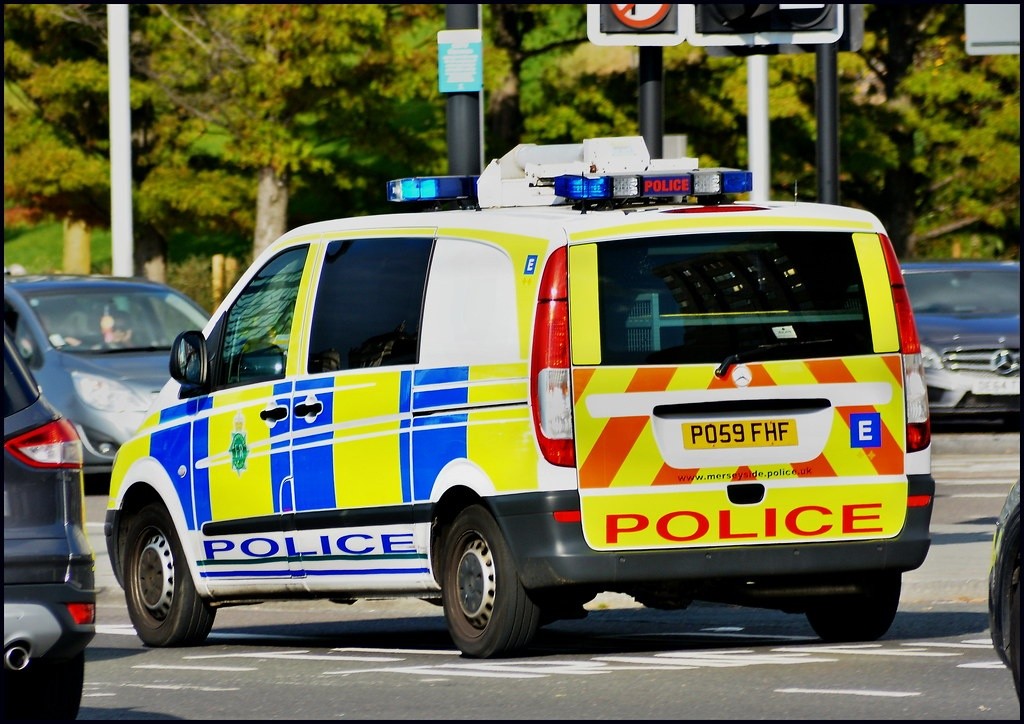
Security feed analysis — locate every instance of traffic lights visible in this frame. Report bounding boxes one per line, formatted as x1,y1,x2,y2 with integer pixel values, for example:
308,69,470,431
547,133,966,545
685,0,842,45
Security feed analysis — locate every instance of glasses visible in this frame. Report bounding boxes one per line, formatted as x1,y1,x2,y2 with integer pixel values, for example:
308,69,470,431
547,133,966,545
104,324,130,333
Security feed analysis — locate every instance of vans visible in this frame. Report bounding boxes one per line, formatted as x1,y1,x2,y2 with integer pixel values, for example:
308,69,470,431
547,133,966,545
104,137,937,659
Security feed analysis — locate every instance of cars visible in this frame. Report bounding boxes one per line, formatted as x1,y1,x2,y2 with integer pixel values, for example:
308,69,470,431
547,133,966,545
2,271,217,491
898,261,1022,433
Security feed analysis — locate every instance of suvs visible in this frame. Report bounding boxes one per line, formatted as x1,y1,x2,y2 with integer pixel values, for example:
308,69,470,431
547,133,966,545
3,318,97,721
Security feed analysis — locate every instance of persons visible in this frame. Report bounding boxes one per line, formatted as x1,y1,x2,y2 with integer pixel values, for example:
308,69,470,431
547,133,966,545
20,312,90,359
100,310,135,349
